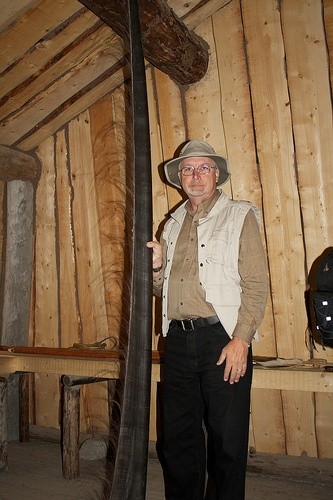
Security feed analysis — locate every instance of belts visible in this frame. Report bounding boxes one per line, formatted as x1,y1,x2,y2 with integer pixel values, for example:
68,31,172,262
170,315,218,331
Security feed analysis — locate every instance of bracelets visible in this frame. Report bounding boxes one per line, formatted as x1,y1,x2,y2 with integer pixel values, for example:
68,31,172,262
151,265,162,273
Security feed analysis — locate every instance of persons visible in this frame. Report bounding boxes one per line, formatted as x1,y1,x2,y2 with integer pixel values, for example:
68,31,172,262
146,140,270,500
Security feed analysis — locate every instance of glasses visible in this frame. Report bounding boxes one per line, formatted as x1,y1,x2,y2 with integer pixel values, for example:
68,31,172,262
178,164,217,176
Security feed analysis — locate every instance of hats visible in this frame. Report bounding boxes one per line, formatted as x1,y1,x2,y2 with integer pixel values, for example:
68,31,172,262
163,140,231,188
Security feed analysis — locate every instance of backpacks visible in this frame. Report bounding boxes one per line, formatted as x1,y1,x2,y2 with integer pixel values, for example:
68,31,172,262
308,245,333,353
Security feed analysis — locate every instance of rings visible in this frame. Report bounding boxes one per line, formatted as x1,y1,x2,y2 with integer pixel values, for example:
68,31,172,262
237,369,243,372
